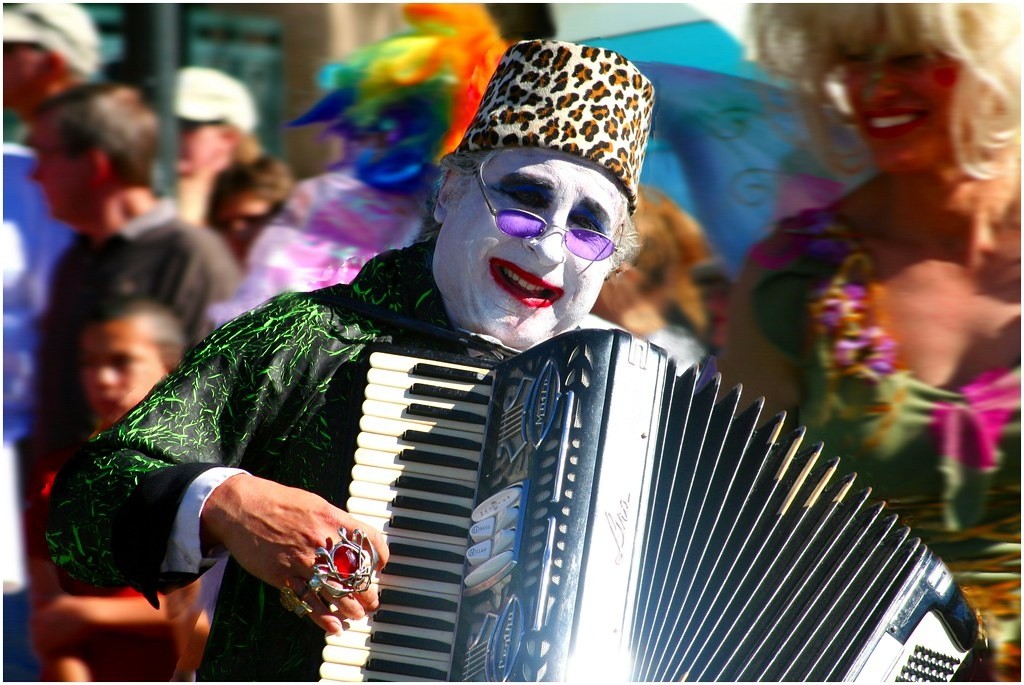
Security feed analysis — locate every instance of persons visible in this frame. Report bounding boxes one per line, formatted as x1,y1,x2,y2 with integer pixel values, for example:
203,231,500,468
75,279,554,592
719,3,1021,683
44,39,995,682
3,3,721,683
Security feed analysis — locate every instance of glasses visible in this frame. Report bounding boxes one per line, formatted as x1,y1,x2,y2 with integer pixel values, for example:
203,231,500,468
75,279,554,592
472,161,624,261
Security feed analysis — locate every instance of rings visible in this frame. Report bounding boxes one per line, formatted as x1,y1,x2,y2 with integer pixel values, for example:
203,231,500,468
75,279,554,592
308,526,380,600
279,585,319,615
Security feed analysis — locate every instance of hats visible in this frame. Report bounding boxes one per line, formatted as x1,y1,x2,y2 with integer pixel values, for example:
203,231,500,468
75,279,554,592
173,67,258,132
3,2,101,73
453,37,657,215
